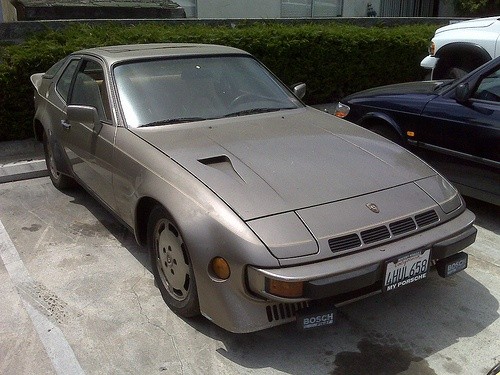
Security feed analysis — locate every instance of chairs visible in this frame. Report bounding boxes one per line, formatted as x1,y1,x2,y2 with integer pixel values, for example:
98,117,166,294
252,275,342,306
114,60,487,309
100,65,228,121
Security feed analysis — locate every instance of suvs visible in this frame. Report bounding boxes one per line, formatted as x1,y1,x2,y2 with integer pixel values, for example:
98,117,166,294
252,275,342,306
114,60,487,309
419,16,500,81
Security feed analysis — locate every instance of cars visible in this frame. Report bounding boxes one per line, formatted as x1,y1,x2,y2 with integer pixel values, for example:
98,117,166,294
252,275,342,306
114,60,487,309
332,56,500,207
30,43,477,335
